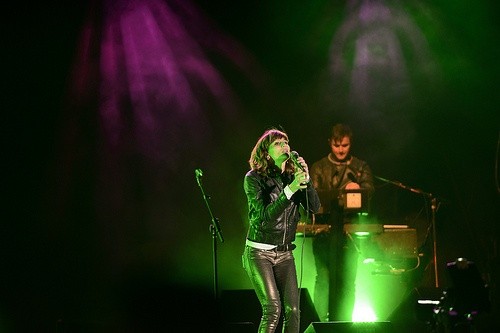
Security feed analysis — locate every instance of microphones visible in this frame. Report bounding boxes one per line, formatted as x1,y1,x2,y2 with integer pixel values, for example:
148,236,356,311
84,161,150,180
348,172,361,186
291,151,306,173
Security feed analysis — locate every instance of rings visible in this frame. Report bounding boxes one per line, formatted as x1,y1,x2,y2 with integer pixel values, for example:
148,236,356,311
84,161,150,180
295,181,298,183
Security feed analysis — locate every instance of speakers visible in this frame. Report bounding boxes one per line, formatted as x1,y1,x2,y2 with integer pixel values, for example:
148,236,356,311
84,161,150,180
304,321,392,333
221,288,321,333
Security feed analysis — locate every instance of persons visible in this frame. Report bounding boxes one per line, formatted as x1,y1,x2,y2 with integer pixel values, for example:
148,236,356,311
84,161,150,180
306,124,375,322
242,128,320,333
